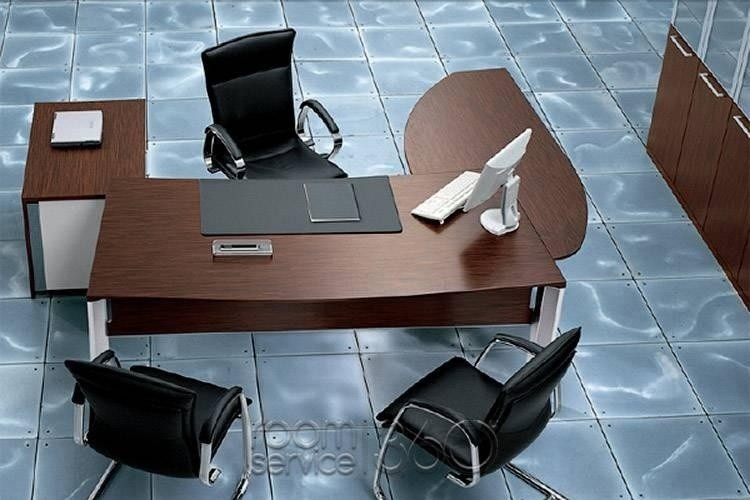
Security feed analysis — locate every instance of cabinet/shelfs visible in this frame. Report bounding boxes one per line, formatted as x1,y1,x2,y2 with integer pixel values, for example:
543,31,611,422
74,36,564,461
699,96,750,282
675,55,732,223
736,231,749,312
646,23,697,187
669,0,749,122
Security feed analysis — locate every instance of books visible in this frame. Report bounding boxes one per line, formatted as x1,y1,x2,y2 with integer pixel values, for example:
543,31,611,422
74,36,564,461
303,183,361,223
50,109,104,146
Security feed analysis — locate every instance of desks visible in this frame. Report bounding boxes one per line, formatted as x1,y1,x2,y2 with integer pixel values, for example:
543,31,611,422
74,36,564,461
77,175,568,365
19,94,145,299
404,62,590,263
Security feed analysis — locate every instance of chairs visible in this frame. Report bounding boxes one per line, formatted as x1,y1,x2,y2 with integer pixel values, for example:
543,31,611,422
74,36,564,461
196,28,354,183
57,342,255,500
366,323,586,500
